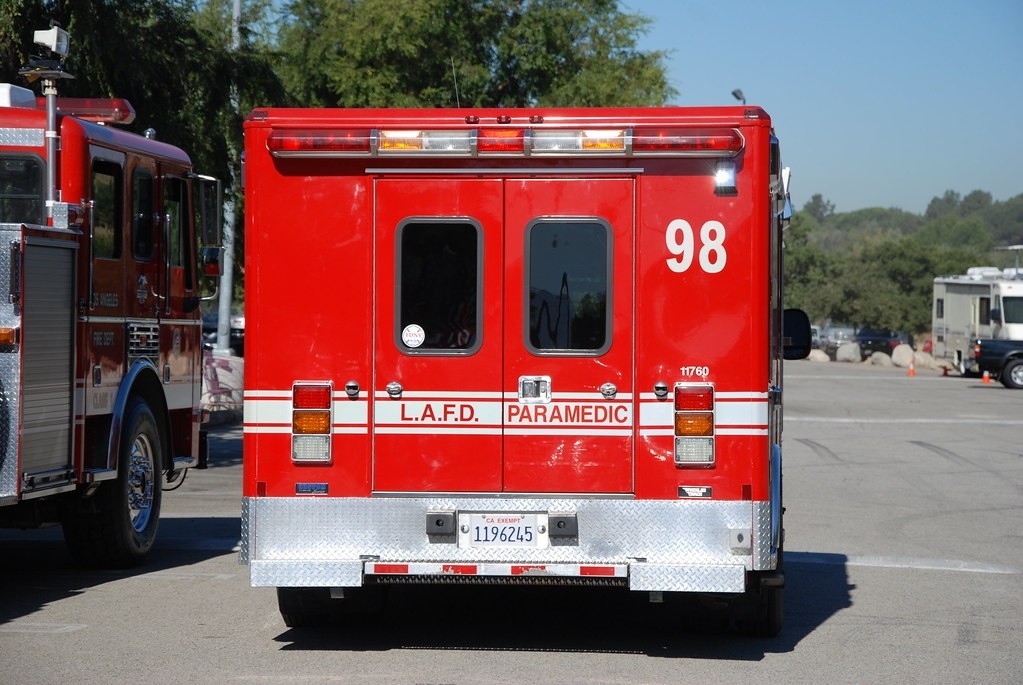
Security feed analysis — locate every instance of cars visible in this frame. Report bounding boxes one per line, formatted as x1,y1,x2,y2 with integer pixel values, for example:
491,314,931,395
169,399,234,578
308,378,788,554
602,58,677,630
200,311,245,426
811,324,917,363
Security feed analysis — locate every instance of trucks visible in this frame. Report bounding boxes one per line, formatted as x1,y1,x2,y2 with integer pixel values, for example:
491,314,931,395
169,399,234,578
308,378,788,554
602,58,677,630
931,244,1023,377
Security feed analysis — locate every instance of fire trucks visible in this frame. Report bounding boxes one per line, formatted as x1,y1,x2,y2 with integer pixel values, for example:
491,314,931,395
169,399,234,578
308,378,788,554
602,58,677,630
0,80,227,571
232,89,813,638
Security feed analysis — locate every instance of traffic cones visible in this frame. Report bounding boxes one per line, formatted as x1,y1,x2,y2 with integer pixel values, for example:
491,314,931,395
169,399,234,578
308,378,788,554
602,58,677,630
905,363,917,377
942,366,948,376
980,369,993,384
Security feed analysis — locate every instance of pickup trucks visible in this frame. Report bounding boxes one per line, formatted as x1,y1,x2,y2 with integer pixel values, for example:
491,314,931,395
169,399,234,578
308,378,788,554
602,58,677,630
969,336,1023,391
